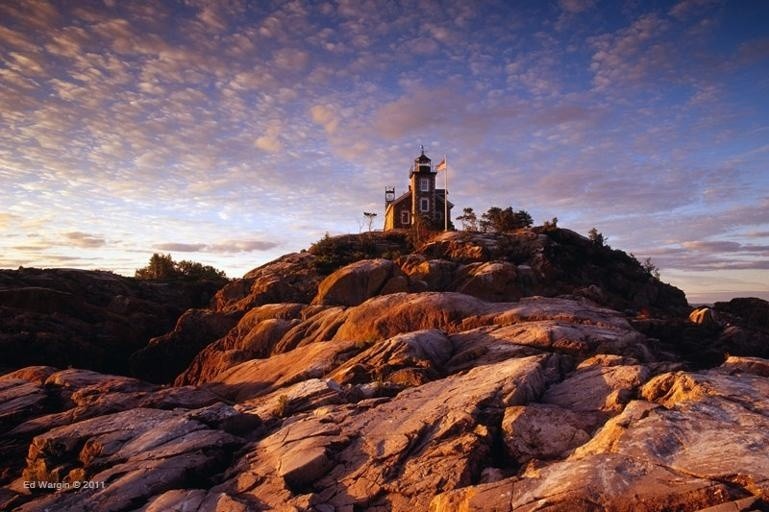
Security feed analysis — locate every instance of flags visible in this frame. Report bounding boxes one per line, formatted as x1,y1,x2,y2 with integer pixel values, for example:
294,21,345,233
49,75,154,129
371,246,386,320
435,160,446,171
